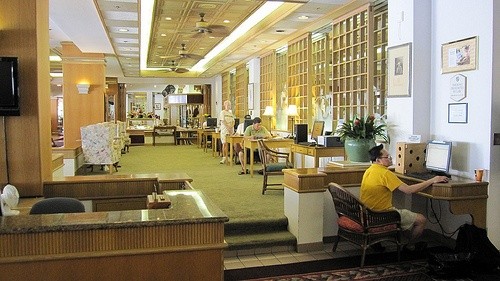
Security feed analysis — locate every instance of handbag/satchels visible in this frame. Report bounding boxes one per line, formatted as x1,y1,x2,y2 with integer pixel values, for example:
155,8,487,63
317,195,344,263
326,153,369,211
455,223,500,274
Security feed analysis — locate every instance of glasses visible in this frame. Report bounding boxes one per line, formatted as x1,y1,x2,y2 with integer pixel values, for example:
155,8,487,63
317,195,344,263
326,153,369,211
378,154,393,159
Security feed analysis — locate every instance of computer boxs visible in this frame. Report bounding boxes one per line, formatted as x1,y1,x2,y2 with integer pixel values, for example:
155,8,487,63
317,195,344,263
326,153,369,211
395,143,429,174
294,124,307,144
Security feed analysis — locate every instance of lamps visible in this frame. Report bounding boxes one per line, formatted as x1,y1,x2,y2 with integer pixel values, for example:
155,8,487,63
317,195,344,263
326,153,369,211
76,84,90,95
286,104,300,139
262,106,276,138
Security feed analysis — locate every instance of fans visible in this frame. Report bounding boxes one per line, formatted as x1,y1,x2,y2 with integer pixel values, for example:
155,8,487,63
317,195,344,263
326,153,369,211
170,13,230,39
157,61,189,73
159,44,204,62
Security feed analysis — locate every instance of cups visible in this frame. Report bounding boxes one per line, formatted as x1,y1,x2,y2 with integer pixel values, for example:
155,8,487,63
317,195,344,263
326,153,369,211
215,128,219,133
476,169,483,184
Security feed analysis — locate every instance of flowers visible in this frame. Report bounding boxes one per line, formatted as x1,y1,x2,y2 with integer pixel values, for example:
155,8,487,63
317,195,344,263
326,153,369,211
333,113,390,146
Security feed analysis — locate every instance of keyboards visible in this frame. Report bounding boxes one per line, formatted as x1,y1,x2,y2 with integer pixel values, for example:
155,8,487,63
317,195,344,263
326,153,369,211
299,142,316,147
205,128,215,129
409,172,436,180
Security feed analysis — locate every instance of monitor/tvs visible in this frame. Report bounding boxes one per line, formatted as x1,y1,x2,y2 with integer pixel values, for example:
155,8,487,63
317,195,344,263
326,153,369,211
311,121,325,141
244,119,254,132
234,119,239,129
207,118,217,128
0,57,21,116
425,141,453,177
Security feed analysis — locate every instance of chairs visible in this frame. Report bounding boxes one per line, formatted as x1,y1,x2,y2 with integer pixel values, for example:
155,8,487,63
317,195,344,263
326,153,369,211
257,138,293,195
327,181,403,267
80,119,131,172
29,197,85,215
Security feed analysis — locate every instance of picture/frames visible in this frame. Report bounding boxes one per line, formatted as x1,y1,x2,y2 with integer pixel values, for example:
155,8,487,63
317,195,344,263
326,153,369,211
441,35,479,75
155,103,161,110
387,43,412,99
448,103,468,124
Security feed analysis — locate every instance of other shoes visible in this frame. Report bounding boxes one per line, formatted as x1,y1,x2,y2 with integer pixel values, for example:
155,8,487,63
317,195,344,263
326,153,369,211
240,168,250,174
402,242,427,254
369,241,387,252
220,156,228,164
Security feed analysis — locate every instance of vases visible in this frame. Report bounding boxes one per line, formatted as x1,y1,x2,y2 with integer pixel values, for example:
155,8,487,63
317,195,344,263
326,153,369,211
347,138,376,162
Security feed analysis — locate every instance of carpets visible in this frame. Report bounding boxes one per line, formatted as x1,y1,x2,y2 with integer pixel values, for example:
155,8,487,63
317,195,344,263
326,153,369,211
240,258,473,281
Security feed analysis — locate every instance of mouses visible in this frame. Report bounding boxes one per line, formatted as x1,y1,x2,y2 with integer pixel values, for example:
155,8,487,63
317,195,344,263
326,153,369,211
440,180,448,183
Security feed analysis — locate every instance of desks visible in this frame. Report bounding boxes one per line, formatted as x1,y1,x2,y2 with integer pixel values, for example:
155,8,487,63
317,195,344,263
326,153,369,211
290,143,346,169
390,169,489,230
197,128,294,178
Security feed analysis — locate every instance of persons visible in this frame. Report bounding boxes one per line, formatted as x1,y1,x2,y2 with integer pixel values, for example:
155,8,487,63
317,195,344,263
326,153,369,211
238,117,272,173
203,115,212,128
359,144,449,251
219,100,235,164
234,114,251,155
456,45,470,65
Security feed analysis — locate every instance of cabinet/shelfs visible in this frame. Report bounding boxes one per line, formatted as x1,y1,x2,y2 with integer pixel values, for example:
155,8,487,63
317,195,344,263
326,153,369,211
152,125,198,146
